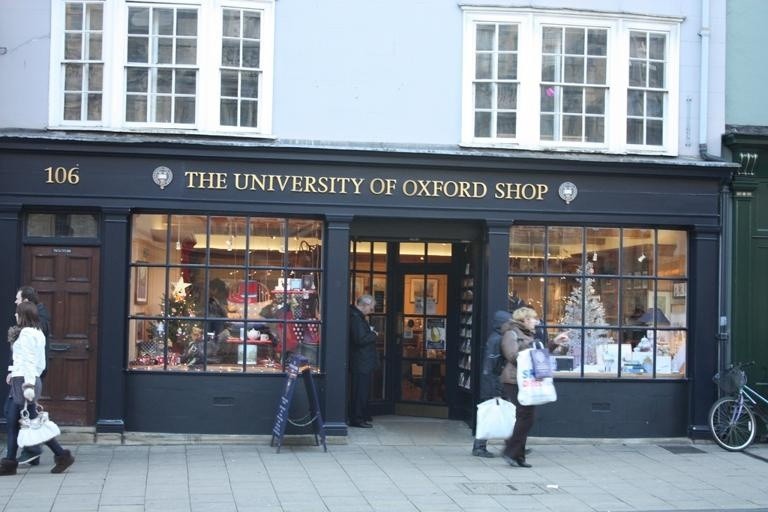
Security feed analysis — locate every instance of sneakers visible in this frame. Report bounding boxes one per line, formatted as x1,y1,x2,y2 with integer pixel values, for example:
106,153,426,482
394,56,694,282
502,452,532,467
17,451,41,465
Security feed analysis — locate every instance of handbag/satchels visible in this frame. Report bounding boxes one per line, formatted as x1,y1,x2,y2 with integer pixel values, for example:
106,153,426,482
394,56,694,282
531,341,553,378
17,399,61,449
490,353,507,375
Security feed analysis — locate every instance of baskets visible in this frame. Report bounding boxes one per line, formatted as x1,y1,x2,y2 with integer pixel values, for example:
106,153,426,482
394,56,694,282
712,366,742,394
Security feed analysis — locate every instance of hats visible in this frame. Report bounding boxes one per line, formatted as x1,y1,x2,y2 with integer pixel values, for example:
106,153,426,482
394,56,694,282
494,311,512,321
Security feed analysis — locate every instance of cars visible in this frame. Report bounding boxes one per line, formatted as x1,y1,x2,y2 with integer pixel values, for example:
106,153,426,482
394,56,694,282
211,276,279,349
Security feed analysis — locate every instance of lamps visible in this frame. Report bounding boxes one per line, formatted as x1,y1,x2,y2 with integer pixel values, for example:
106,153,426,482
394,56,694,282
636,307,671,340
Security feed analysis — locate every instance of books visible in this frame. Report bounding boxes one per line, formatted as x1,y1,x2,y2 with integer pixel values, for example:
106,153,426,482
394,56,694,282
457,263,474,391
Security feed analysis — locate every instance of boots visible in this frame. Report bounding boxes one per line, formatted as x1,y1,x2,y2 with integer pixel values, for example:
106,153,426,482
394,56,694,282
473,438,492,457
52,450,74,473
0,458,18,475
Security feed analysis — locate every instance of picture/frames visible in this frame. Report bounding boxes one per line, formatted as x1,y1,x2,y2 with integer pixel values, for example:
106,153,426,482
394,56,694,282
135,260,149,345
409,278,439,304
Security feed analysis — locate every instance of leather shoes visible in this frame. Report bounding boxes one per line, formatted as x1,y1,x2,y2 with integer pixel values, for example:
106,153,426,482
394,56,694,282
350,419,372,428
364,415,372,421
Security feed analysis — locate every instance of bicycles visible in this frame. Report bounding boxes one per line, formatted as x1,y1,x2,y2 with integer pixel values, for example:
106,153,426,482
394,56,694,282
707,359,768,451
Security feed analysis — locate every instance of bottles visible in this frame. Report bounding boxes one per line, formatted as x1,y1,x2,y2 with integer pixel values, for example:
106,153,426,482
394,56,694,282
239,327,244,342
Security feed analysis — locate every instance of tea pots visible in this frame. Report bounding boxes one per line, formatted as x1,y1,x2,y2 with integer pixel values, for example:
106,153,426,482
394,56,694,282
246,327,261,340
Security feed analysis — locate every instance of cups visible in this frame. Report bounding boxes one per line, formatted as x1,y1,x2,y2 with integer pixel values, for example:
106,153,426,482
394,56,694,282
260,333,270,341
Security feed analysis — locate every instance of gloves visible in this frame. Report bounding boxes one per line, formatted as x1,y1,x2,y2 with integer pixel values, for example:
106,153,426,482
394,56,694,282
528,338,544,348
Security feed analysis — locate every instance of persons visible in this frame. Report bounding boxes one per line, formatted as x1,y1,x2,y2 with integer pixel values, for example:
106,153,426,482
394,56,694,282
350,293,379,429
192,277,281,364
14,286,52,465
499,306,573,469
621,303,649,351
471,310,513,458
0,301,75,475
